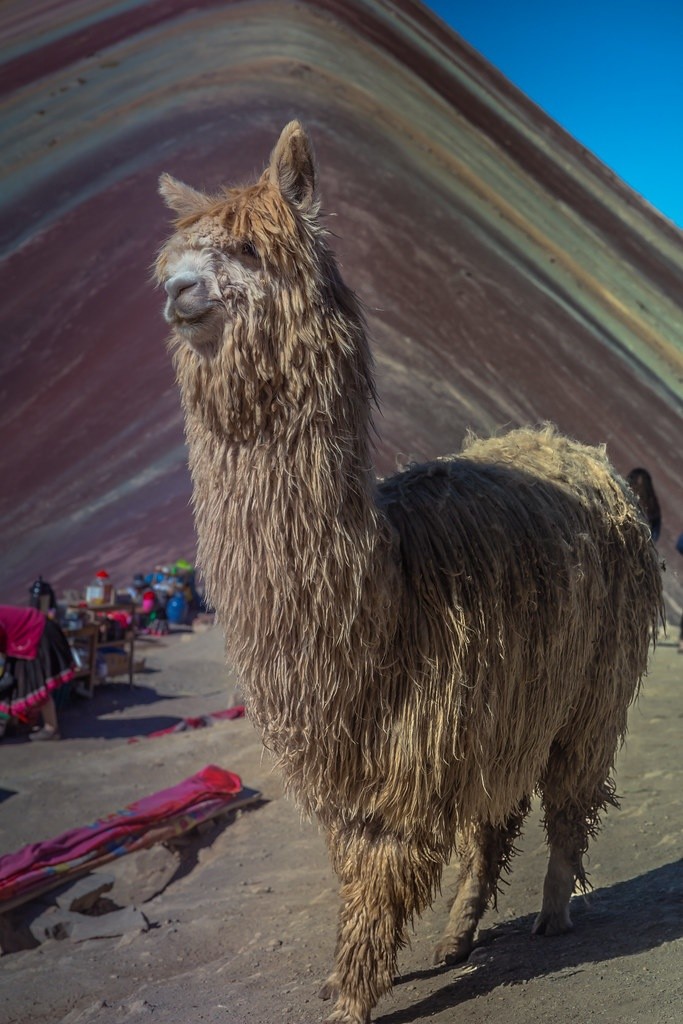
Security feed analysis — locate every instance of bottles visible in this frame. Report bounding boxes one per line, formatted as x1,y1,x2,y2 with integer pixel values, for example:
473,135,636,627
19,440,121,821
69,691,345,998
89,569,112,607
166,591,187,624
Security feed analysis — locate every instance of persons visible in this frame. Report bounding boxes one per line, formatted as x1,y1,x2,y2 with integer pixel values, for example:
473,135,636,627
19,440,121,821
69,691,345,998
0,604,81,742
626,467,662,542
131,573,170,635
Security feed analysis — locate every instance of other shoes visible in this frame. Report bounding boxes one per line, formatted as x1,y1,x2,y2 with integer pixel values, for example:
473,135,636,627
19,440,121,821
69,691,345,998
28,727,62,741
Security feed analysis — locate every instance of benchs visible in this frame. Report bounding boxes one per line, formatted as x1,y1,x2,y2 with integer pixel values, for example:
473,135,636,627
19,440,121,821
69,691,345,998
58,599,138,699
